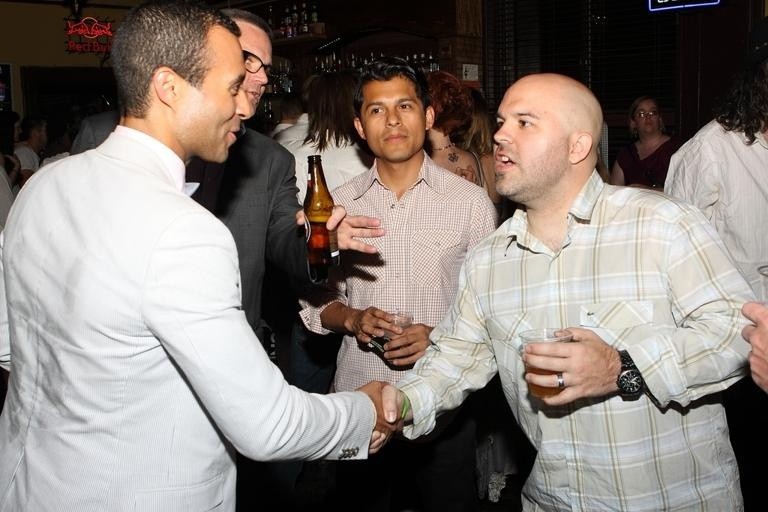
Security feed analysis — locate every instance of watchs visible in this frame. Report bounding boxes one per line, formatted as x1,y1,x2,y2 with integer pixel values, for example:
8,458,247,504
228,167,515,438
615,350,642,397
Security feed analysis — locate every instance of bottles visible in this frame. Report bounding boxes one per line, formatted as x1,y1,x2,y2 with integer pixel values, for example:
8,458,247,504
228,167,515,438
286,8,292,38
305,155,339,303
405,54,410,62
280,13,285,36
419,53,426,69
291,4,298,37
301,3,308,33
264,100,267,115
411,54,418,69
427,52,434,72
267,6,277,37
268,99,273,122
311,4,318,22
312,51,384,72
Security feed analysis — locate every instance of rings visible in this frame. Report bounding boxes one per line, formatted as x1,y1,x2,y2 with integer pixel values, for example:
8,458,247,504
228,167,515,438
557,374,564,389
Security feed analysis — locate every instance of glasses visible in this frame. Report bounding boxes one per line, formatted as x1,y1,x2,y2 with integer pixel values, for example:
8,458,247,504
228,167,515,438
633,110,658,117
243,50,278,84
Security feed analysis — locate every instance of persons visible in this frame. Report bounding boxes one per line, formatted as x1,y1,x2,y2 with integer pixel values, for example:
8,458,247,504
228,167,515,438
420,70,522,505
666,18,766,511
0,2,496,511
612,99,683,197
365,82,758,512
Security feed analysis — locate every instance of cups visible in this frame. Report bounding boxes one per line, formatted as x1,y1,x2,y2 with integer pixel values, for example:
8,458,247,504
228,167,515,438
758,266,768,307
518,328,572,398
384,313,413,370
